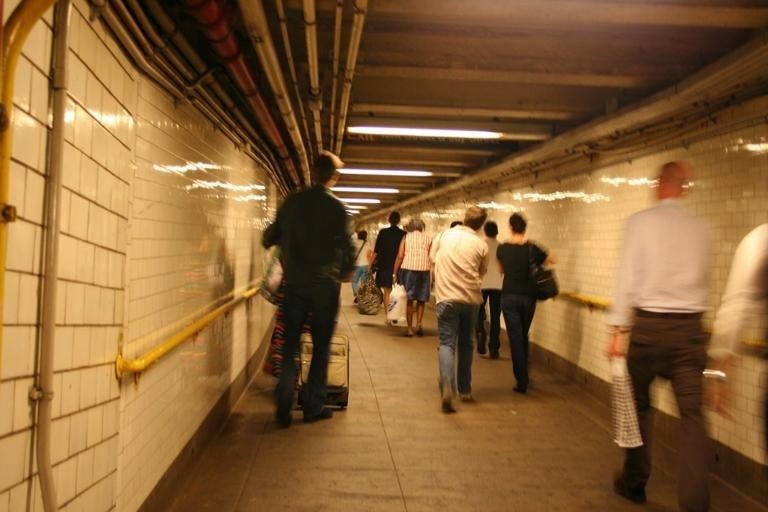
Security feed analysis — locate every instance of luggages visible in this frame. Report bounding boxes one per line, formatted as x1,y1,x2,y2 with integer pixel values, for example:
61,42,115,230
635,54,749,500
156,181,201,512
300,333,349,408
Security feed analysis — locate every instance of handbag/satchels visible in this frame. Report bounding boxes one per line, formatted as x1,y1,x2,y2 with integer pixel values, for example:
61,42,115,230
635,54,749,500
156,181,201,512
533,269,559,300
388,283,408,327
357,281,383,314
611,356,643,448
257,257,284,306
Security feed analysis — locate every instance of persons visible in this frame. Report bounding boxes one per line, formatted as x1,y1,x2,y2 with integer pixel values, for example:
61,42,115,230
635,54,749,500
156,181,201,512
351,208,435,337
428,206,551,413
710,222,767,511
261,151,357,429
607,162,712,512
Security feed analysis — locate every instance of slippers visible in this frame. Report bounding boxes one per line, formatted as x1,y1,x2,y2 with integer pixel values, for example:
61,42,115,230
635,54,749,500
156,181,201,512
406,328,423,336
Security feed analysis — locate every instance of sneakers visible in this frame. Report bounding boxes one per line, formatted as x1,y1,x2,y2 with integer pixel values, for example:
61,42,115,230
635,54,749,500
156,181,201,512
612,474,646,504
459,393,473,401
513,384,527,393
305,408,333,422
476,332,499,359
278,410,293,428
442,396,456,413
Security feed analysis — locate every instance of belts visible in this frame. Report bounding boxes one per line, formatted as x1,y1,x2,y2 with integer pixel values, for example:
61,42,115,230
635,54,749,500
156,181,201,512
634,308,703,318
